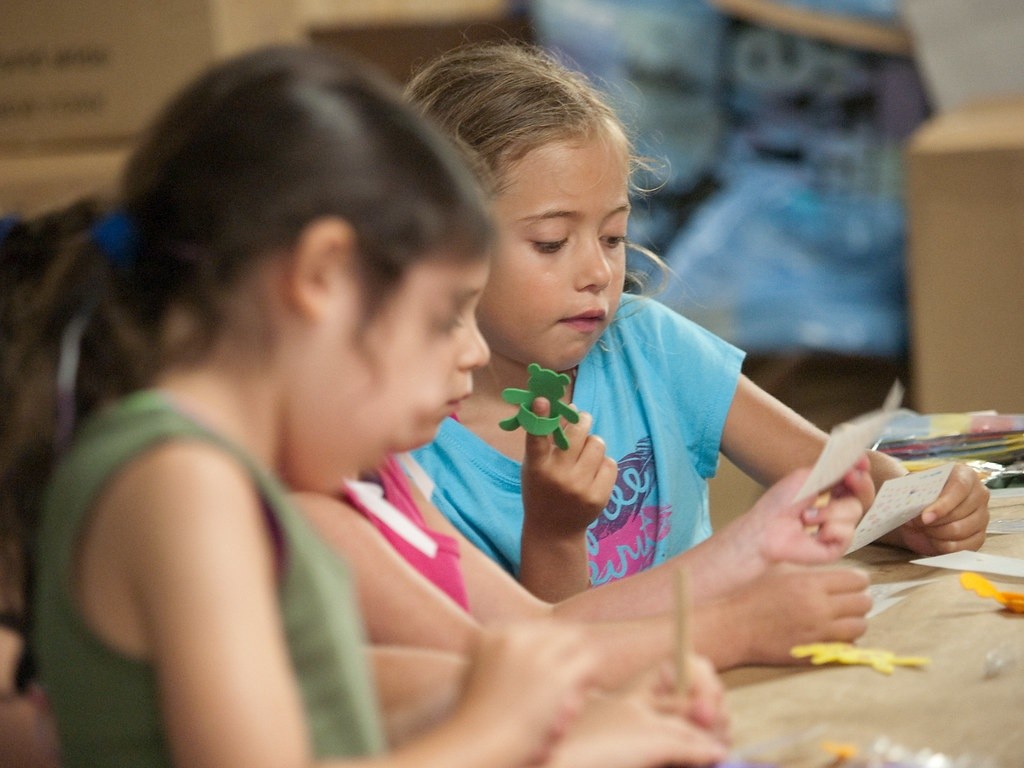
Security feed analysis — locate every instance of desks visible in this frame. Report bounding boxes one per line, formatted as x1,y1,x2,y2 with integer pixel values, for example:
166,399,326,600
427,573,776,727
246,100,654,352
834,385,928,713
706,452,1021,764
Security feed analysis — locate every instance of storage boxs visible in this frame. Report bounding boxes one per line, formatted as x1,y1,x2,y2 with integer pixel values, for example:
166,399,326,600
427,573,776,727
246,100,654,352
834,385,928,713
904,95,1023,416
0,0,316,227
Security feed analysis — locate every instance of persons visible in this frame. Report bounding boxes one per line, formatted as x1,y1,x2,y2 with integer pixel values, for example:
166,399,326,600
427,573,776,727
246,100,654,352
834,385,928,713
392,40,988,611
1,40,730,768
270,249,874,679
